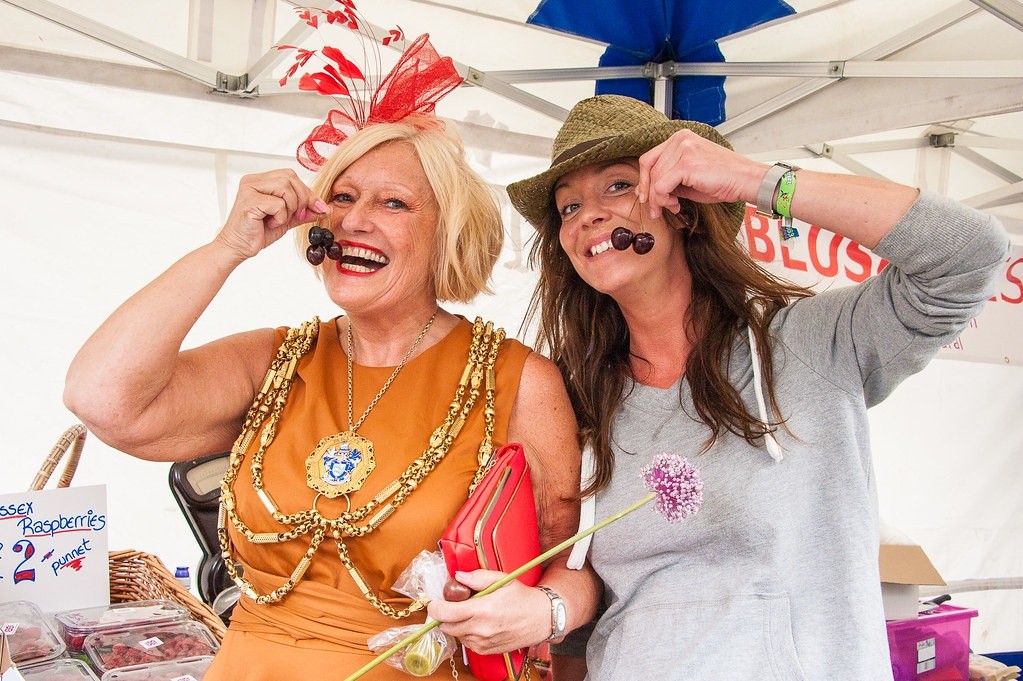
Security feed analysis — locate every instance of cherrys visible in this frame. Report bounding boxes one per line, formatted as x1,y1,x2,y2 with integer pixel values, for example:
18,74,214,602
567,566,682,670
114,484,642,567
306,213,342,266
611,195,654,255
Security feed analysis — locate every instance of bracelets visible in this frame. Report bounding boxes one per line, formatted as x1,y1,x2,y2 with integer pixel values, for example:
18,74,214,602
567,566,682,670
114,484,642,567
754,162,800,249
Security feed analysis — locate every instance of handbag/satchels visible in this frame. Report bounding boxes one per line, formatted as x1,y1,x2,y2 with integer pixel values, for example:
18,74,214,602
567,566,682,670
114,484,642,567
441,442,544,681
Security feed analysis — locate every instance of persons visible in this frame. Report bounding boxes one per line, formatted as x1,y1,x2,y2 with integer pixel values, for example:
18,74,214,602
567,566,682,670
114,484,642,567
63,110,602,679
504,94,1012,679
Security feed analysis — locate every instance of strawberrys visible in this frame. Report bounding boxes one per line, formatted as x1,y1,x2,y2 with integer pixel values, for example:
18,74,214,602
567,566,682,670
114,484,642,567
64,616,173,650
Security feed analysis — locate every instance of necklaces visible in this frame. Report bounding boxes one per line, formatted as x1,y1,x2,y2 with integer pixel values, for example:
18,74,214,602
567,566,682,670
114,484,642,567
217,312,507,620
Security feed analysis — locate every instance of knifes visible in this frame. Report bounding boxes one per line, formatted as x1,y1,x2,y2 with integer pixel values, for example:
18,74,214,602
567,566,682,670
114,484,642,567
919,594,951,612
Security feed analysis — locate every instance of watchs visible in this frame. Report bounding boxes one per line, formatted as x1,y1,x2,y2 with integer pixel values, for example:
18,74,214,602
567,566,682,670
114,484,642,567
535,585,567,641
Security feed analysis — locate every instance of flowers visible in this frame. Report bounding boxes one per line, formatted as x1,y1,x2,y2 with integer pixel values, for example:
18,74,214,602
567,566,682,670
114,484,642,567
343,452,704,681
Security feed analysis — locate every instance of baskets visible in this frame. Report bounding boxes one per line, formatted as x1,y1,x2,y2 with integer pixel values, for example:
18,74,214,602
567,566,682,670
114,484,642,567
28,424,228,648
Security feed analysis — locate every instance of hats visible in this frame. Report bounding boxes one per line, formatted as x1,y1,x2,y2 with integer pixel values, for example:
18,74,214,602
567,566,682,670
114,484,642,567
506,94,746,251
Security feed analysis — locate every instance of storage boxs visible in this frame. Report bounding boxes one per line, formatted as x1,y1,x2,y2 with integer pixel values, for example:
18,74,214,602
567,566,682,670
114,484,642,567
101,654,215,681
53,598,191,655
81,620,221,677
878,542,947,622
18,658,100,681
886,602,979,681
0,599,67,667
